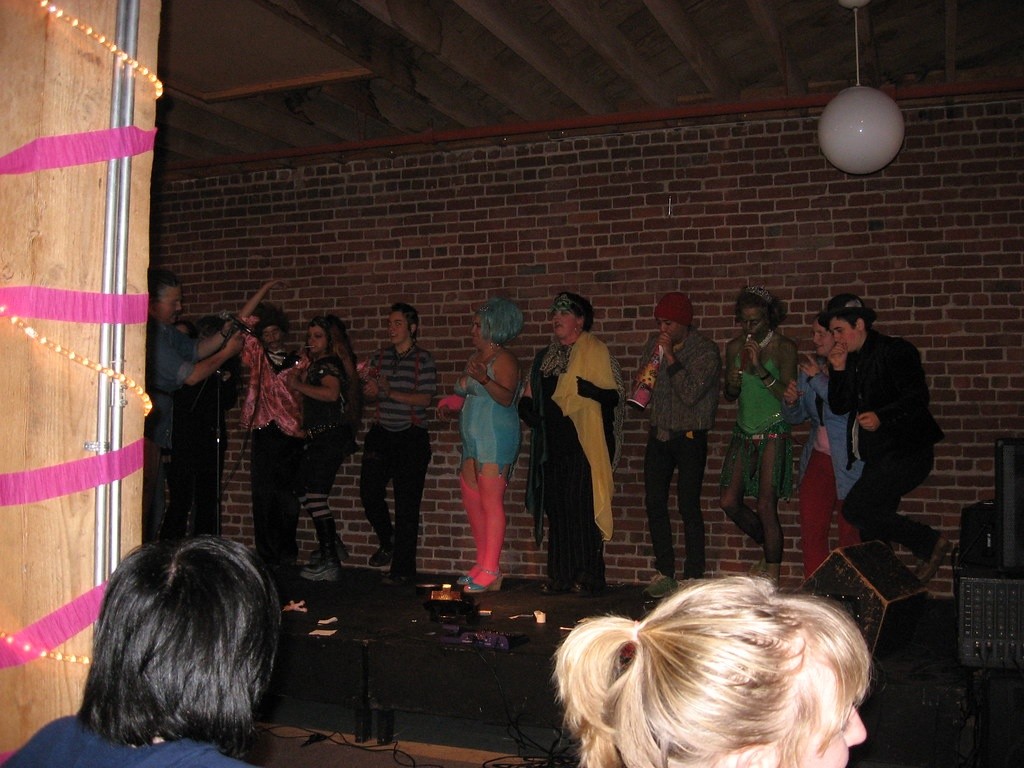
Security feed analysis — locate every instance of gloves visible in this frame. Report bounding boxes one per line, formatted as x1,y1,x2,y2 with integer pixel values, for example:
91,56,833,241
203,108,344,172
518,396,540,428
576,376,620,407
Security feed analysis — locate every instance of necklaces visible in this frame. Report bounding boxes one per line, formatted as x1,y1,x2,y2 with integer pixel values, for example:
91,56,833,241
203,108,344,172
389,344,415,374
758,328,774,349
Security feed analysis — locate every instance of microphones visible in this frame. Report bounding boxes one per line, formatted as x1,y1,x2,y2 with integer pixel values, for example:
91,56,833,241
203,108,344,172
218,309,252,334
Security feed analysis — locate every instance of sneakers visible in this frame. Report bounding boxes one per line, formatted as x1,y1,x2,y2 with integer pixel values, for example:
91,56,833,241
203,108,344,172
642,571,677,599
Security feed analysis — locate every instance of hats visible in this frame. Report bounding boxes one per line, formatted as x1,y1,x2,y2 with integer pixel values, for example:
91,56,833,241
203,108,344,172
654,292,693,325
817,293,877,325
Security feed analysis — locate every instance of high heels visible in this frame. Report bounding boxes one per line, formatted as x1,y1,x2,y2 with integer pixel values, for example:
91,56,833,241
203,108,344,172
457,563,503,593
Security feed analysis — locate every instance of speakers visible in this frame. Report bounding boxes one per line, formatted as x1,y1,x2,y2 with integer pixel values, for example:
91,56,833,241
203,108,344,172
787,539,928,660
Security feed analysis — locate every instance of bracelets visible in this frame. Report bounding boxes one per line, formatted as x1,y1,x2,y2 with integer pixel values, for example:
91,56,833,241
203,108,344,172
767,378,777,389
760,372,772,381
480,374,490,386
219,329,228,340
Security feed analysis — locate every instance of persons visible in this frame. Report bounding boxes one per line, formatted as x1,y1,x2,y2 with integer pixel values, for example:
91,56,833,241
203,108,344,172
281,314,362,582
234,279,311,584
516,291,626,597
625,293,724,604
0,533,281,768
147,266,256,543
554,573,872,768
776,291,954,595
359,302,437,600
719,285,800,590
438,299,524,594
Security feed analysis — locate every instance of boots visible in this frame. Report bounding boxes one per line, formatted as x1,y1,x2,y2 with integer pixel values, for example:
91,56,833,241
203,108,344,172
300,517,349,582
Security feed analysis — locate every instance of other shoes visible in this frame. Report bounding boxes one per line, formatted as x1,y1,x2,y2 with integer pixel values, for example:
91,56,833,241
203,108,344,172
367,545,394,568
576,579,607,596
917,535,953,582
541,581,572,595
747,544,780,588
383,577,415,586
